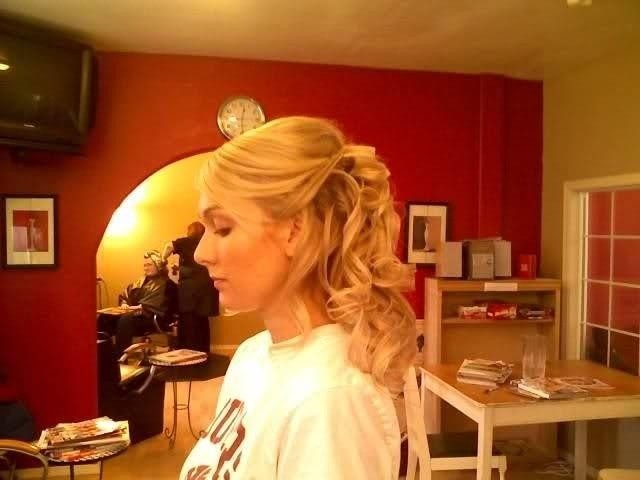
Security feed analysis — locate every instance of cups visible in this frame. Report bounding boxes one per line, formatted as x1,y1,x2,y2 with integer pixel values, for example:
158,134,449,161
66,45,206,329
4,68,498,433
521,335,547,379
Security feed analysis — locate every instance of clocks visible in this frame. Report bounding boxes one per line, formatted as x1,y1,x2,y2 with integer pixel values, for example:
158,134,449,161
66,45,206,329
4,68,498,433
217,94,267,142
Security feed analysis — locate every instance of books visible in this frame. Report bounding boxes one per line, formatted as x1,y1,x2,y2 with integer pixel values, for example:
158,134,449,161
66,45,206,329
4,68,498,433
145,348,208,363
148,357,207,367
34,415,133,465
96,306,137,316
456,356,584,400
433,236,539,280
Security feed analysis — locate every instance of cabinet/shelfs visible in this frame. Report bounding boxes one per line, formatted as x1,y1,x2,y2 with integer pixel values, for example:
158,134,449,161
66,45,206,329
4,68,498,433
424,277,561,459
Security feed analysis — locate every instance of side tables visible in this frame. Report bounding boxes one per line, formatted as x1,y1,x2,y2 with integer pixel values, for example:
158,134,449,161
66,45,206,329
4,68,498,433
147,352,230,449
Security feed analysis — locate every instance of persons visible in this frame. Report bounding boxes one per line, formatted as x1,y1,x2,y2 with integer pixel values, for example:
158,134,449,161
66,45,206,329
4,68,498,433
169,116,422,479
162,220,220,352
96,248,177,352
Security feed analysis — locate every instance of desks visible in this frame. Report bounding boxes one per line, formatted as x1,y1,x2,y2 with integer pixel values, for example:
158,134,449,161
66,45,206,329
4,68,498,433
419,360,640,480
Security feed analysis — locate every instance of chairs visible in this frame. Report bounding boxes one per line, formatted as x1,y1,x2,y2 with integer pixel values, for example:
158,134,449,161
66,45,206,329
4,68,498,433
404,366,507,480
96,331,165,445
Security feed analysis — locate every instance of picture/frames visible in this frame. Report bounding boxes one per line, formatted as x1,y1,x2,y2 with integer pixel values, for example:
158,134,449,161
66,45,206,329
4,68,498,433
405,201,450,268
3,194,58,269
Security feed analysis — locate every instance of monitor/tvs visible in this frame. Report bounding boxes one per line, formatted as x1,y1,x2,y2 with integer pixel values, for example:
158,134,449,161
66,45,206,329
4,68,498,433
1,17,99,154
